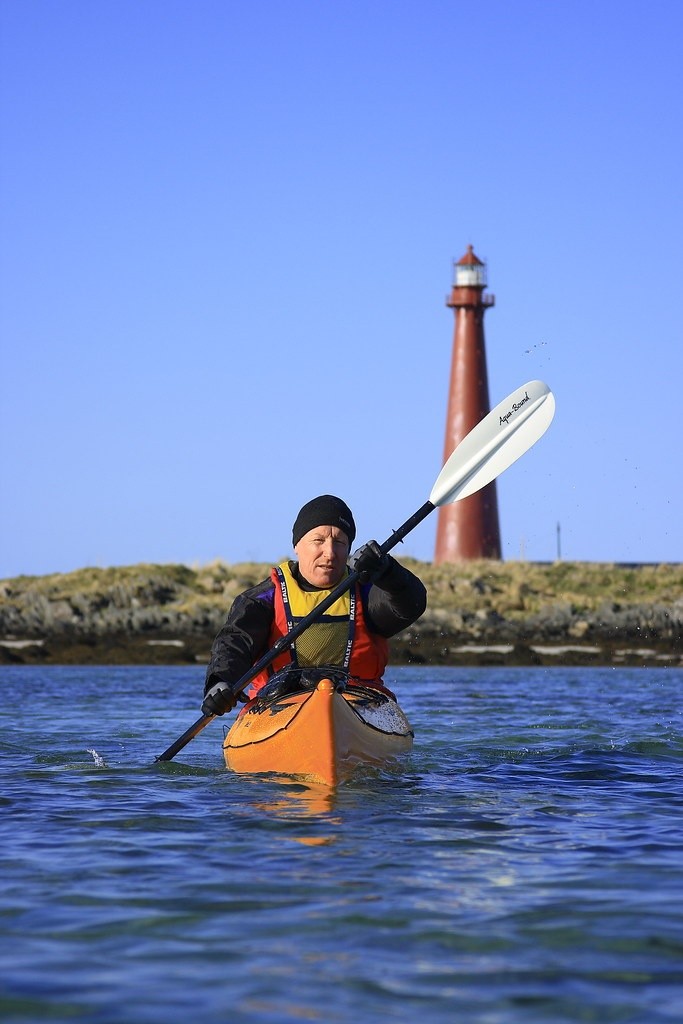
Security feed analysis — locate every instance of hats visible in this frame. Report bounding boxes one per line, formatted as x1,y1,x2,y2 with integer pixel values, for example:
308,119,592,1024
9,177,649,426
292,495,356,551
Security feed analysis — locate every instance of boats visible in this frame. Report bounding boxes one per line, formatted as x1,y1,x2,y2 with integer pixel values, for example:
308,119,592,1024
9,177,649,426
222,679,417,786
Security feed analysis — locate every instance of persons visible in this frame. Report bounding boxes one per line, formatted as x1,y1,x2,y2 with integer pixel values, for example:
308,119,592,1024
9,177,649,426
200,495,427,720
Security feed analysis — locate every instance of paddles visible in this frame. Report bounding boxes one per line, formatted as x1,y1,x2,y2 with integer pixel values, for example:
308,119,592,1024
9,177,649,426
156,380,558,762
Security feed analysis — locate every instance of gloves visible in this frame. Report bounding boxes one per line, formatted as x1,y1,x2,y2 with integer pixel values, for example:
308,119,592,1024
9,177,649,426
200,681,250,717
346,540,389,586
